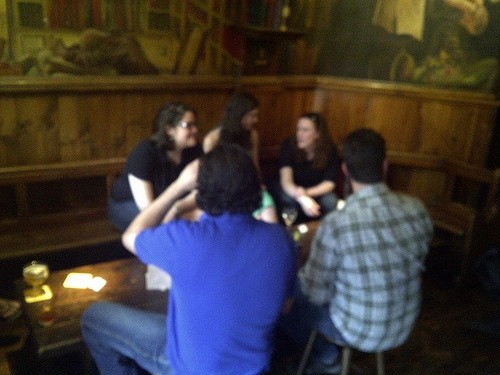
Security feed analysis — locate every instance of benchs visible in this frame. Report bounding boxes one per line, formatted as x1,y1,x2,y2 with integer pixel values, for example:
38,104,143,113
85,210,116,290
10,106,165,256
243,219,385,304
0,144,500,270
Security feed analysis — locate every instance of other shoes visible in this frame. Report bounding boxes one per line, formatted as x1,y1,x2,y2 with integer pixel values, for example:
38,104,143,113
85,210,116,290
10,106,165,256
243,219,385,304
311,358,341,373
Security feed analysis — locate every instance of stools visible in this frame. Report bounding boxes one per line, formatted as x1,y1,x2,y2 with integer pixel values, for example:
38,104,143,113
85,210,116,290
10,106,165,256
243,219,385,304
296,331,385,375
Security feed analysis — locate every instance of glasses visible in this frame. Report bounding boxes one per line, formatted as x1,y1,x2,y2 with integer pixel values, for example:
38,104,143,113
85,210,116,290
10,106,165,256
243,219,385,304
177,121,198,128
305,113,320,128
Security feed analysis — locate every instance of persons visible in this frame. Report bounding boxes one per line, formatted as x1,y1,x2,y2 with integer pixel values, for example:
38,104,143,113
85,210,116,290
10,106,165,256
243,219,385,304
201,92,278,225
80,141,296,374
276,127,432,375
107,102,210,228
277,111,340,217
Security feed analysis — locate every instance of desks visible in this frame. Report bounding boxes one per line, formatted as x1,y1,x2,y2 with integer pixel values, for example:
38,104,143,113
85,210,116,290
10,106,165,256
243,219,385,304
23,222,319,355
